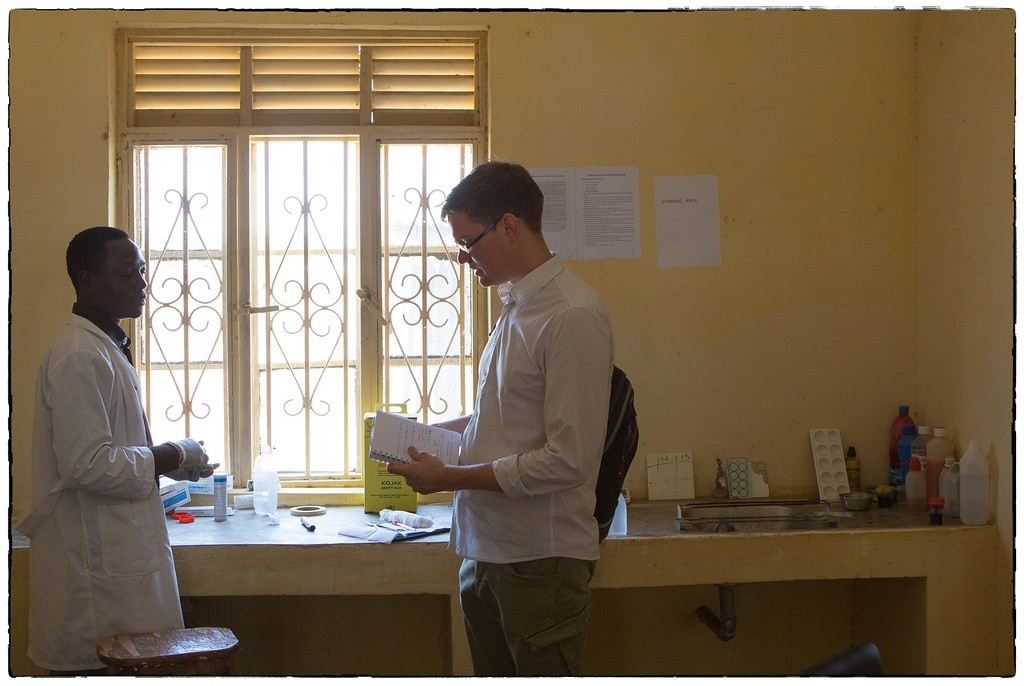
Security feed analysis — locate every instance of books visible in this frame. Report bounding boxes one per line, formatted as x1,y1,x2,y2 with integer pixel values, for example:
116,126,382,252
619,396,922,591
369,410,462,466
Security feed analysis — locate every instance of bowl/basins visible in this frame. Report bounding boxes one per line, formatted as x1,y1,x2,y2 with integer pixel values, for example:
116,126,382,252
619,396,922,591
840,493,873,511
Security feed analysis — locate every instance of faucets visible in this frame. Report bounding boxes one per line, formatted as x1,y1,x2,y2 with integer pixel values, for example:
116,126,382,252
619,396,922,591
712,457,729,499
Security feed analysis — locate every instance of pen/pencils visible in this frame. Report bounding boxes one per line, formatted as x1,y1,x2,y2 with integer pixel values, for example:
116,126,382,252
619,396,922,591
377,525,393,531
301,518,316,531
394,521,415,530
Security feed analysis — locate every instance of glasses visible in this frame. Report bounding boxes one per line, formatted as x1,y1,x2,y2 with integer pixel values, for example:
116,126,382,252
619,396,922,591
453,220,501,254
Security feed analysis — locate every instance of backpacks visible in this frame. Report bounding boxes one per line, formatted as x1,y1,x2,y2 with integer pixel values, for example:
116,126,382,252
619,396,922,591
593,364,639,548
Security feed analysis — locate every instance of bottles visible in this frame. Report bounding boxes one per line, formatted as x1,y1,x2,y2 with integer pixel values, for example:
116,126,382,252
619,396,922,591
846,447,861,492
911,425,932,459
929,496,945,525
898,427,919,478
939,456,960,515
960,440,990,525
905,456,927,511
889,405,915,485
926,427,954,499
253,446,278,515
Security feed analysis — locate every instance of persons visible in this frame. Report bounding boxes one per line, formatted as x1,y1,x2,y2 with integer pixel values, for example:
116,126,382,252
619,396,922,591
27,227,220,677
388,161,614,678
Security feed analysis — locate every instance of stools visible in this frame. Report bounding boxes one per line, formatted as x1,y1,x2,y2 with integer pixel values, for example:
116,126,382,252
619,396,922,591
95,625,240,677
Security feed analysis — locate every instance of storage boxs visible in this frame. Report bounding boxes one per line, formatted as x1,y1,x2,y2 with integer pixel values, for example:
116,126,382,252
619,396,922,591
363,412,417,513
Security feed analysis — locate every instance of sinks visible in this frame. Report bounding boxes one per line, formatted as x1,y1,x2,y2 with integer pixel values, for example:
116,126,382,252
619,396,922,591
675,500,842,534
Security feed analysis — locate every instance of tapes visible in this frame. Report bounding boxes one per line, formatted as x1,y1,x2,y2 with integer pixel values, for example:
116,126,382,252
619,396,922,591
290,505,326,516
